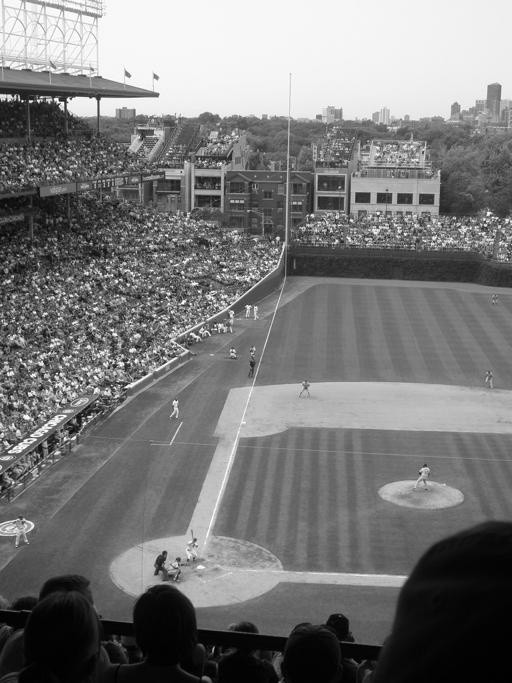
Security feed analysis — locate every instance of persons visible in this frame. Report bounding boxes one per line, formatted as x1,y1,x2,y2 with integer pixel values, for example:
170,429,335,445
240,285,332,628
290,209,512,262
415,463,430,490
372,520,510,682
486,370,495,388
154,537,198,580
317,129,425,178
297,380,310,399
492,295,498,303
1,575,371,680
0,96,284,502
14,516,28,548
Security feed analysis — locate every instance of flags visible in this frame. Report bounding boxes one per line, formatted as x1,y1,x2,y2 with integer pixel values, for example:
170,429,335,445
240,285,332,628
125,70,131,78
154,74,160,80
90,67,94,71
50,61,56,69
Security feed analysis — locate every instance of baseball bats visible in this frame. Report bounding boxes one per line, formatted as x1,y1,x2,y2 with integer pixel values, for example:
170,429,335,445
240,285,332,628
191,529,195,545
